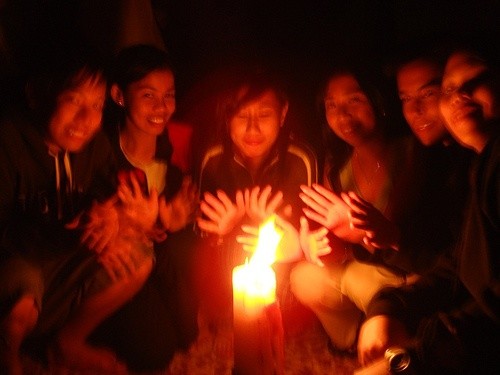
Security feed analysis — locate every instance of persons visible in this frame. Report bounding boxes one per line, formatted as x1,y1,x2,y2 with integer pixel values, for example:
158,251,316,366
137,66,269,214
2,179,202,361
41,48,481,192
0,42,500,375
